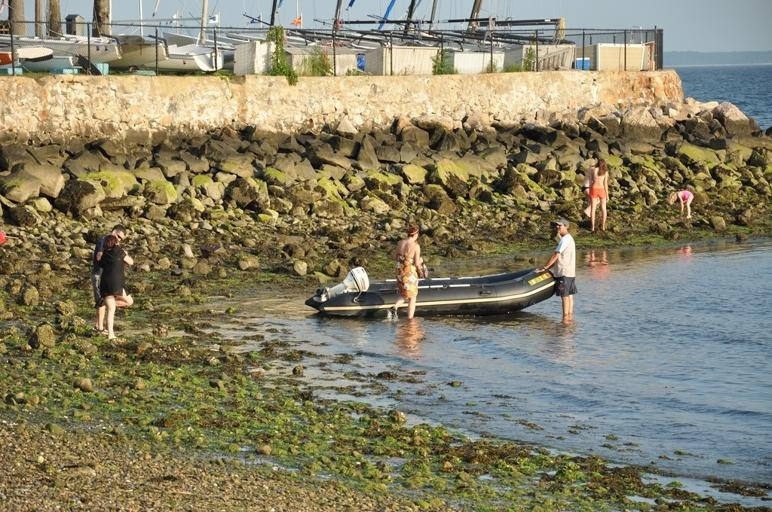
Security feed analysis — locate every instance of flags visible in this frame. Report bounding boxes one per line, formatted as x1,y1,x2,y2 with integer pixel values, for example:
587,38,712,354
290,16,302,25
208,14,218,23
165,13,177,25
247,16,260,23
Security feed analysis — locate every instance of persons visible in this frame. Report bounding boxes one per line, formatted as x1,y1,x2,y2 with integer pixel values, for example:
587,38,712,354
588,248,611,280
669,190,694,219
589,158,610,234
388,221,424,324
92,223,127,334
97,235,135,339
533,219,577,317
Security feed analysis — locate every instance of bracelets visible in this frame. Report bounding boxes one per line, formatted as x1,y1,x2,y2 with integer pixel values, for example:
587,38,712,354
543,266,547,271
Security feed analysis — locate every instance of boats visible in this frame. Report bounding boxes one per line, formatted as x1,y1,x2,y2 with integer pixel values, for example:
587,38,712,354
304,264,557,318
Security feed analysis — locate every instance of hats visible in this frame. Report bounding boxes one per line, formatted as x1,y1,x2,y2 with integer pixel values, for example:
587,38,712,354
406,226,419,234
556,220,570,228
